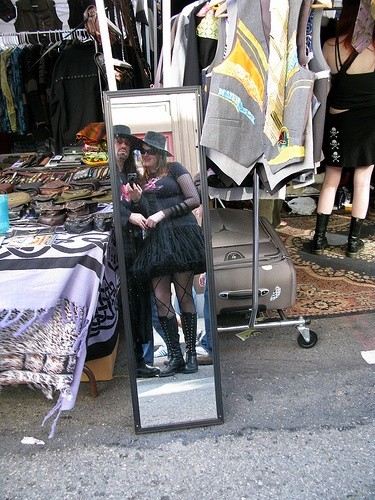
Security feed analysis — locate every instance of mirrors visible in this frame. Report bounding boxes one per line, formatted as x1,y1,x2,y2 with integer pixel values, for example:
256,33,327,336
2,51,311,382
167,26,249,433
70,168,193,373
101,85,227,436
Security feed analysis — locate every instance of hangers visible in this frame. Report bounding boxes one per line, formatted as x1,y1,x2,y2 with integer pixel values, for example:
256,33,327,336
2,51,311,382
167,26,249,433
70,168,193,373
0,29,96,52
180,0,333,18
197,0,332,20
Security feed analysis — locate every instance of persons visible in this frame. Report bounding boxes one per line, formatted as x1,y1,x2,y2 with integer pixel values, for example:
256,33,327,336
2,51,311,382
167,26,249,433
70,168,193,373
113,126,213,378
312,0,375,258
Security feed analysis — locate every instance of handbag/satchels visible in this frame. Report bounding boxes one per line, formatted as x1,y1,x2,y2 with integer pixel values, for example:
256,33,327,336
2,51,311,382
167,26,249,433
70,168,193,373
95,51,136,90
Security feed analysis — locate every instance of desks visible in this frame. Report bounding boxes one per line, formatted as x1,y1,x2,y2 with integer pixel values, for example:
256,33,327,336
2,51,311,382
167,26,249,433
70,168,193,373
1,226,117,398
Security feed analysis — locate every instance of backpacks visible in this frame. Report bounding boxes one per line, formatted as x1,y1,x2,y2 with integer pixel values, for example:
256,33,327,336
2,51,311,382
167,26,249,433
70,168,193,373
84,5,122,47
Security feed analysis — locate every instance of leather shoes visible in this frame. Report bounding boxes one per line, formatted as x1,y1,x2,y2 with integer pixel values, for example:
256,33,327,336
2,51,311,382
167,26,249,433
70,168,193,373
136,364,160,377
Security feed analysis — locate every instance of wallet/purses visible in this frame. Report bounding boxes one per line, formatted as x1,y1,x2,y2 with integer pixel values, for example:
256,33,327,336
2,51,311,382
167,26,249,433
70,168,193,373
0,152,113,233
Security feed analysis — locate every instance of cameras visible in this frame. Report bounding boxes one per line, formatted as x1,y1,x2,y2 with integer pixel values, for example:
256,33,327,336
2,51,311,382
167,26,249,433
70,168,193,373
127,173,137,189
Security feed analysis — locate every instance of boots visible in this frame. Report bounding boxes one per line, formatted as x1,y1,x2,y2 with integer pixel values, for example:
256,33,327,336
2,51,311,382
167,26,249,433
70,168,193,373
181,311,198,374
345,216,364,258
311,212,330,255
157,314,186,376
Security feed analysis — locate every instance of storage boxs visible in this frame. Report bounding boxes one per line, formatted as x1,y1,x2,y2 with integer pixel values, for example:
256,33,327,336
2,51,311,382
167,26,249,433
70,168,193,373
80,333,120,382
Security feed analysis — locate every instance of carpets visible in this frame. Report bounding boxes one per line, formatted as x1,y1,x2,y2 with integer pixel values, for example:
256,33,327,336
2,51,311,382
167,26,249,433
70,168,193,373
245,209,375,319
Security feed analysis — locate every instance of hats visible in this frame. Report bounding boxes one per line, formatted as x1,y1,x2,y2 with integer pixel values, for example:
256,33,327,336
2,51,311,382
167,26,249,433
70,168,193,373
112,125,142,150
141,130,173,157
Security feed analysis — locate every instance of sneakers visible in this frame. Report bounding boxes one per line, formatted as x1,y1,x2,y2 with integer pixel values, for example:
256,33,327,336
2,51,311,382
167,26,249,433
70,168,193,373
198,354,213,366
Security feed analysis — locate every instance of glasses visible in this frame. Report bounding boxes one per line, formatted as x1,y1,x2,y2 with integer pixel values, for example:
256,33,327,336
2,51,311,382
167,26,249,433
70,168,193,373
140,147,158,155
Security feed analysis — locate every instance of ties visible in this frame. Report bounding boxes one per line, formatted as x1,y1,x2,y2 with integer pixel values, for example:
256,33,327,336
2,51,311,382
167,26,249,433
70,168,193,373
263,0,289,146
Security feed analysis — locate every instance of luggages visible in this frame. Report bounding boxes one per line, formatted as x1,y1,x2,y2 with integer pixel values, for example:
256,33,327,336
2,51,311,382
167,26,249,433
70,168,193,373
208,208,297,317
